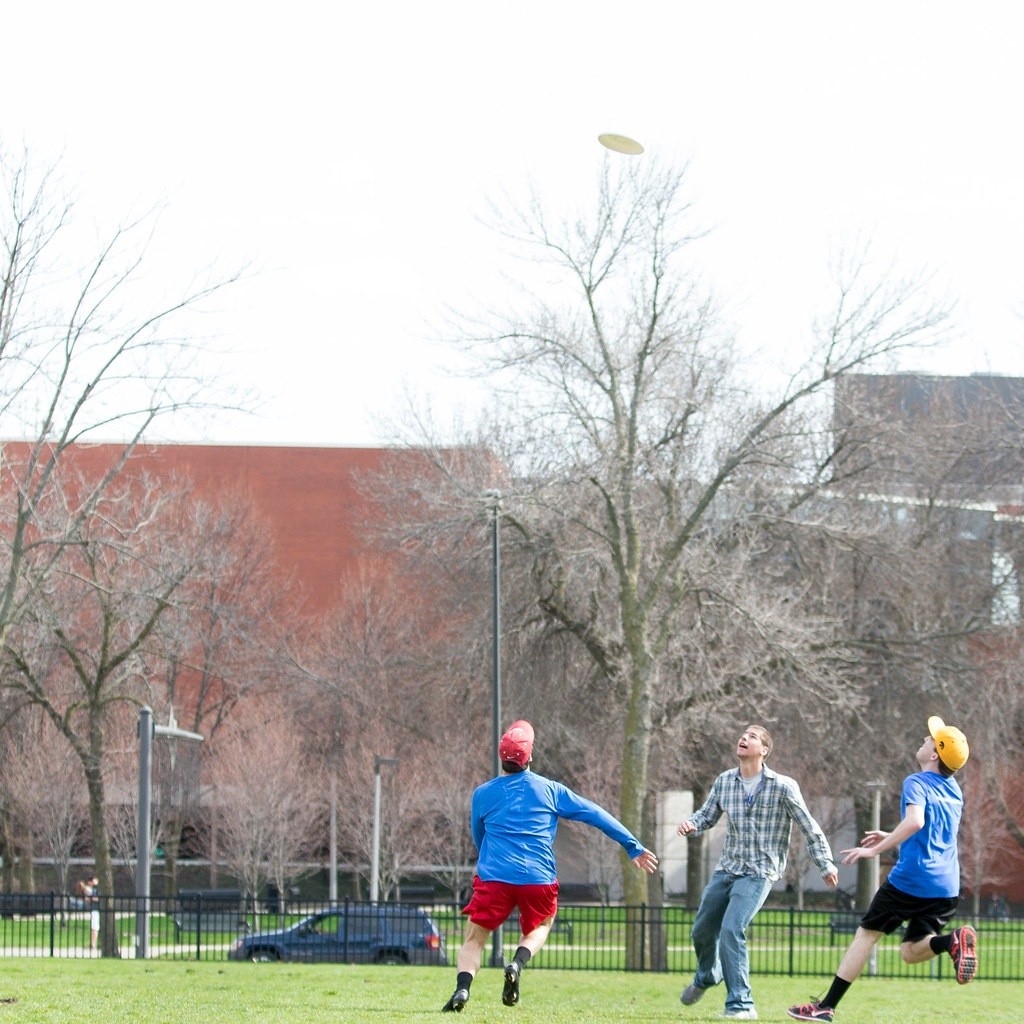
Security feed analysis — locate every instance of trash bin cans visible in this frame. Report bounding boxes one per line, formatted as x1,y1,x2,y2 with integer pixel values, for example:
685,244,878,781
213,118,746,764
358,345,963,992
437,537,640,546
266,883,281,915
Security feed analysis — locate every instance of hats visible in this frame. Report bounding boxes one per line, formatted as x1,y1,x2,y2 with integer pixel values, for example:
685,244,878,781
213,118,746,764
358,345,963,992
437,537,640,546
498,720,535,767
927,716,969,771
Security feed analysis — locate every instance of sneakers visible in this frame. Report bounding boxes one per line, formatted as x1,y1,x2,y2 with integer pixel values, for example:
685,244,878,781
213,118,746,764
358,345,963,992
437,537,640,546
442,989,470,1012
501,962,520,1007
714,1007,758,1020
680,982,708,1006
786,996,836,1022
950,925,978,984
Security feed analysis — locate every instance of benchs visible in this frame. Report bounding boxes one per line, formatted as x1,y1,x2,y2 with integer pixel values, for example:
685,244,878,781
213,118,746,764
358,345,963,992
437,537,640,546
502,911,573,946
829,915,907,947
174,889,250,944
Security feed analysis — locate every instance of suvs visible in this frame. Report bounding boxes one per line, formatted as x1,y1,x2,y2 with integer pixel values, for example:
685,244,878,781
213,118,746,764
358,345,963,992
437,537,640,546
226,903,448,967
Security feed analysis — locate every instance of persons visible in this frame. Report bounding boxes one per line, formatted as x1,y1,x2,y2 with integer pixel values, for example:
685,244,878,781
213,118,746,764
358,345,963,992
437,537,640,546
679,725,838,1015
441,721,659,1012
83,878,100,953
788,716,978,1023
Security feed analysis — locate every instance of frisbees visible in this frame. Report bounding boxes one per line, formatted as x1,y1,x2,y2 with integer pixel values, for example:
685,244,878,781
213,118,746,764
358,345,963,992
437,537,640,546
598,134,645,156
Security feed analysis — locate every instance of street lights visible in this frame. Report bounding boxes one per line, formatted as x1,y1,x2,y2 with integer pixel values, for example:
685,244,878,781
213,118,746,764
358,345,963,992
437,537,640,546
480,487,505,971
